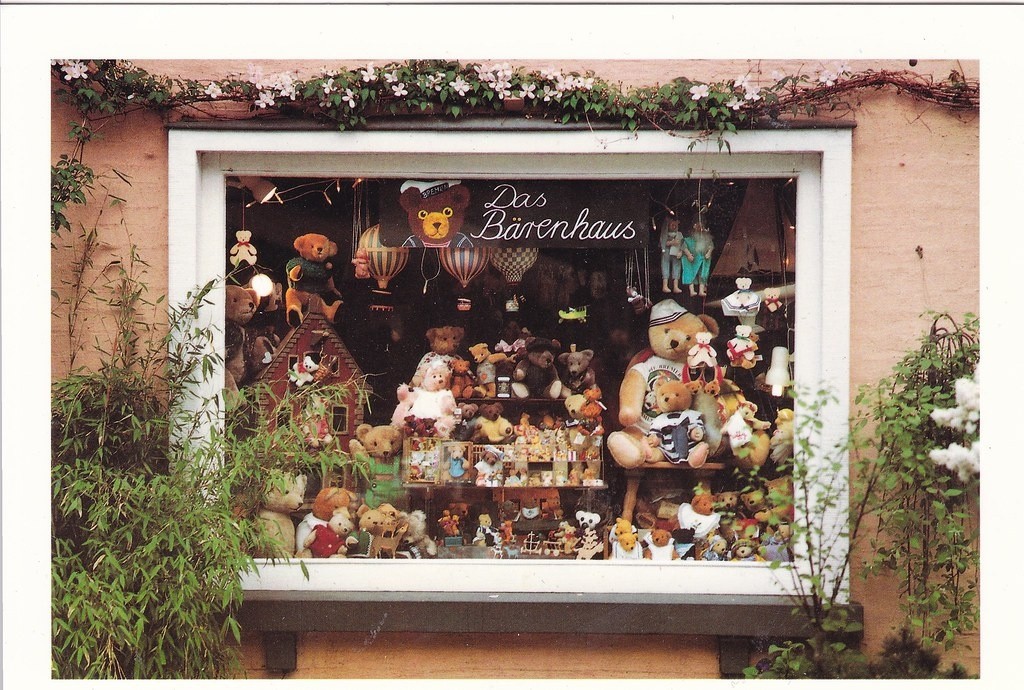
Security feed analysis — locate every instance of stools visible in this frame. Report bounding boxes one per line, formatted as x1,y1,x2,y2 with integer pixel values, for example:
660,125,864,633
612,461,726,560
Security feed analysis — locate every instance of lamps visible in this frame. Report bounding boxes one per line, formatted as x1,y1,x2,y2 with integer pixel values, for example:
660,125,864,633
766,347,790,397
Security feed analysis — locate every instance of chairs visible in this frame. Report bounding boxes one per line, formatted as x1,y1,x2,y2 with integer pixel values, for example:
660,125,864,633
371,522,409,559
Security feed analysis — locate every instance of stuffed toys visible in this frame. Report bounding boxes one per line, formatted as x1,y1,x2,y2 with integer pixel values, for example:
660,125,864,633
226,174,796,561
